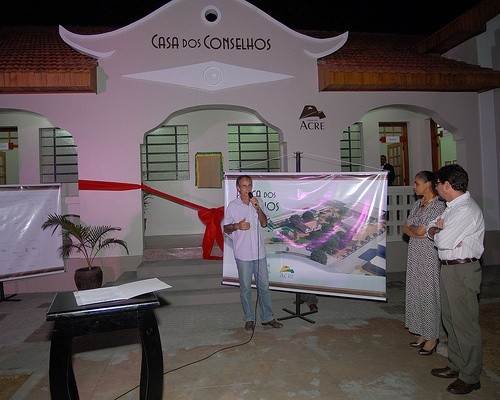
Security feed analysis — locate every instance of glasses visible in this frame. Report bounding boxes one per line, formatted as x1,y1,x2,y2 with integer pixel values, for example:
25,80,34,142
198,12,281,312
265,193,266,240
239,184,253,189
434,181,443,186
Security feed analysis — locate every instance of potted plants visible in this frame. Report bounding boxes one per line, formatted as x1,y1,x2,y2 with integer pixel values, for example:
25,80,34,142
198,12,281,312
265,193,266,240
143,191,153,236
41,213,129,290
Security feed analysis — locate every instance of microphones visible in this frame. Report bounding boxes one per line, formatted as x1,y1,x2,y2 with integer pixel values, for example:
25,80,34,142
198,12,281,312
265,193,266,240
248,191,258,206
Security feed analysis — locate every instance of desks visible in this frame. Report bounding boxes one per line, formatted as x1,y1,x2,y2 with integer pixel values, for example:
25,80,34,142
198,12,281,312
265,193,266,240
45,286,168,400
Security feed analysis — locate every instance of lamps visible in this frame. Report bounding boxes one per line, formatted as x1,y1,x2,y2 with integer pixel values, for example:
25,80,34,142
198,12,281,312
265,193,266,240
437,130,444,137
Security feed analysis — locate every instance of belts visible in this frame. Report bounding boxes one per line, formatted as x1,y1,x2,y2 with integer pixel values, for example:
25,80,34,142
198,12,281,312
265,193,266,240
440,257,477,266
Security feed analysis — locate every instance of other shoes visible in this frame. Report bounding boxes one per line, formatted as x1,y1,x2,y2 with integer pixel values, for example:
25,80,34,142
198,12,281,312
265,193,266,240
261,319,283,329
293,298,306,304
244,321,254,332
309,304,318,312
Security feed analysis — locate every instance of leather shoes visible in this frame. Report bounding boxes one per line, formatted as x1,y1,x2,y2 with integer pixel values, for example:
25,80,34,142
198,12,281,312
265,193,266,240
447,377,481,394
431,366,459,378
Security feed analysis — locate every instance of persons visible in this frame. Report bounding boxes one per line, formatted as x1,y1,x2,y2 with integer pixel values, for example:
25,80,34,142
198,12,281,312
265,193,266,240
222,175,283,331
402,171,446,356
380,155,395,221
426,165,485,394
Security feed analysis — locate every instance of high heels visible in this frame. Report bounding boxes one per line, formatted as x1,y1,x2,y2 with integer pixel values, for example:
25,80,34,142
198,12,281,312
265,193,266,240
409,340,426,348
419,338,439,355
